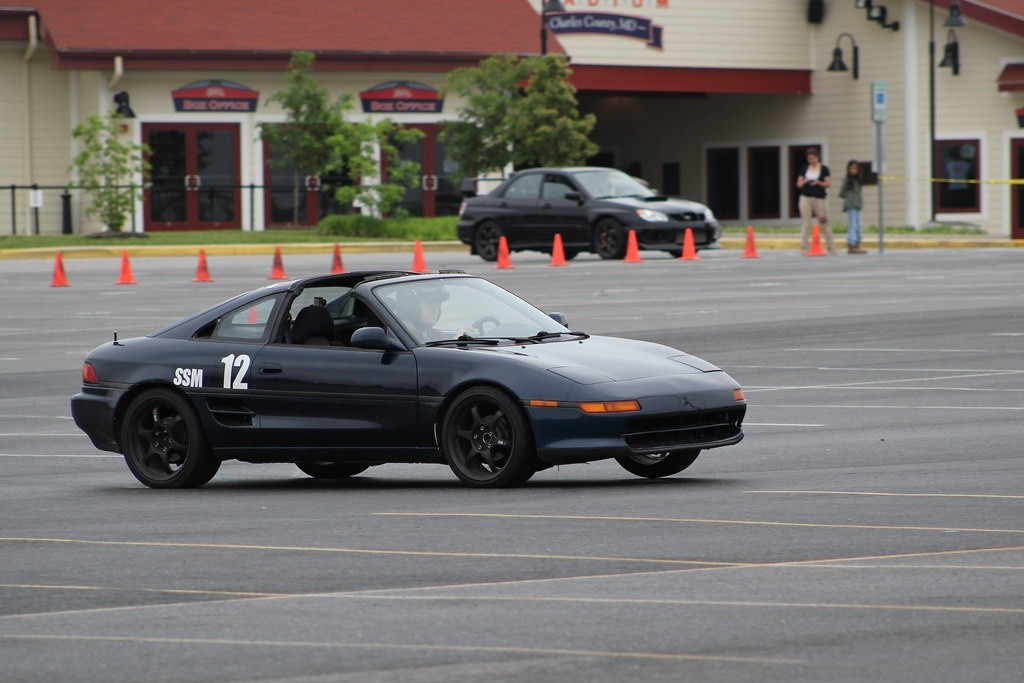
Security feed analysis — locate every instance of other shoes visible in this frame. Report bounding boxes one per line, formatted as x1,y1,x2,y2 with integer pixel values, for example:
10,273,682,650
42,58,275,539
848,248,867,253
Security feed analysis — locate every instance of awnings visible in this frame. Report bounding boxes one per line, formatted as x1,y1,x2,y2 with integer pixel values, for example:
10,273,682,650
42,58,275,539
997,64,1024,92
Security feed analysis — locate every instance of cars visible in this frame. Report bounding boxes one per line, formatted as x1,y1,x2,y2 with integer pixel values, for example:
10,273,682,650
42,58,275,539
72,269,747,488
458,165,720,262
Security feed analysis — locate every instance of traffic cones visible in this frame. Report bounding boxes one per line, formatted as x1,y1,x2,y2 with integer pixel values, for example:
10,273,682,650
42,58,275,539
48,252,71,287
112,252,139,285
328,244,346,273
739,226,761,258
267,246,289,278
190,249,215,282
623,230,644,262
548,233,568,266
804,224,828,256
495,236,514,269
410,240,428,272
675,228,699,260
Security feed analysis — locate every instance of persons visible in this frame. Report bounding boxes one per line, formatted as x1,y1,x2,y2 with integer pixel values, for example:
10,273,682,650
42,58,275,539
392,281,475,343
798,148,837,255
839,159,868,253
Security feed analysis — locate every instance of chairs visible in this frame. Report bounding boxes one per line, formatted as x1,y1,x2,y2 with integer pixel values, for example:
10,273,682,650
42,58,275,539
291,305,339,346
367,297,396,328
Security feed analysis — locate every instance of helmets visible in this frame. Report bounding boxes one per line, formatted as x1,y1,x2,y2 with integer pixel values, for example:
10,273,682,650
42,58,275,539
396,287,450,328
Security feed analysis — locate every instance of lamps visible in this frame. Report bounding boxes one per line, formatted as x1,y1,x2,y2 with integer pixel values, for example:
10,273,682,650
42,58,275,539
828,31,858,79
944,1,967,27
855,0,898,30
113,90,136,119
940,30,962,76
